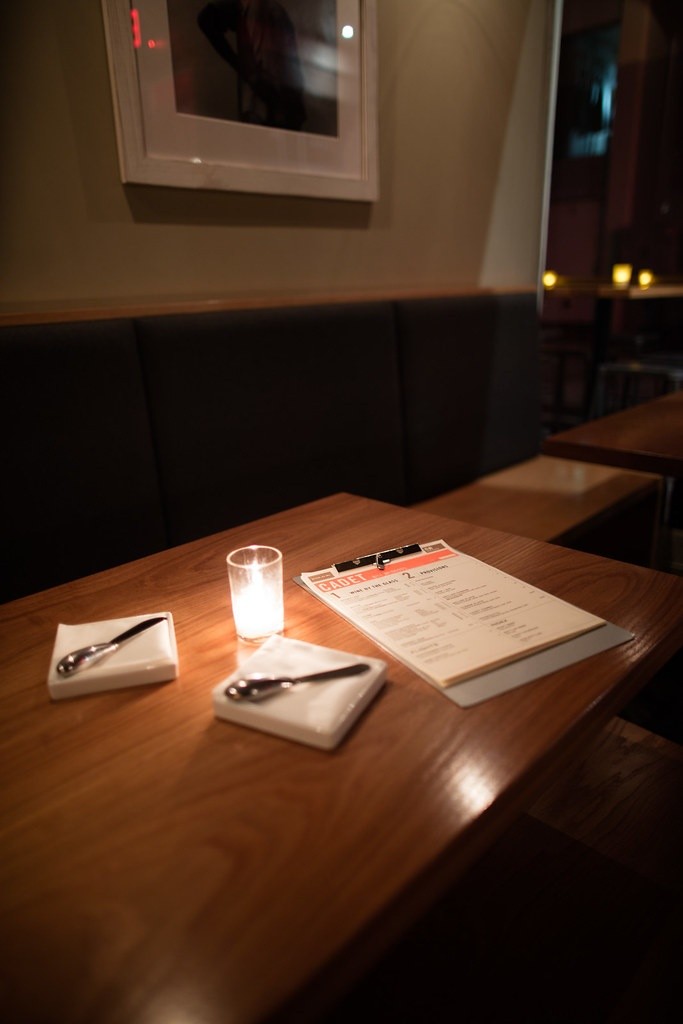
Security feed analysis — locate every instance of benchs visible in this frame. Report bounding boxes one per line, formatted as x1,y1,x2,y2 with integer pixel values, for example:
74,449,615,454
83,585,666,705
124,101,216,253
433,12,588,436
0,289,665,606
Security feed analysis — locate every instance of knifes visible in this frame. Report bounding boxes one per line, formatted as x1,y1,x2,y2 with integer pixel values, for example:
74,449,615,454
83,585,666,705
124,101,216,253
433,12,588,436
57,616,169,676
225,664,370,702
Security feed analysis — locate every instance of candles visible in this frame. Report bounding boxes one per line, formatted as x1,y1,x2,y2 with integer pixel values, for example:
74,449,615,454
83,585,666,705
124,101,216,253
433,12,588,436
225,546,285,641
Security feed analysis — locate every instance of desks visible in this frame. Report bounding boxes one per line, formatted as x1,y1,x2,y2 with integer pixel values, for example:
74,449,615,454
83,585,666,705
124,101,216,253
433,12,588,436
542,389,683,576
599,279,683,411
0,489,683,1024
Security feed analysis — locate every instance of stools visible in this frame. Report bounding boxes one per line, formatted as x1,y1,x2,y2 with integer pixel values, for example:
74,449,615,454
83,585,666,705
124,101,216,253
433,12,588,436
537,316,601,431
590,360,683,419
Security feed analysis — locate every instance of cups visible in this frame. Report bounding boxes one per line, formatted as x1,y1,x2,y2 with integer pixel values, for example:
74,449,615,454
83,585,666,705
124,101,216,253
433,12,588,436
226,545,285,643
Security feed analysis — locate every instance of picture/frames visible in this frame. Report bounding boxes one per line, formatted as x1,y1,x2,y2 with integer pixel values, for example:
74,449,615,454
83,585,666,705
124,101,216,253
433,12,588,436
102,0,379,204
